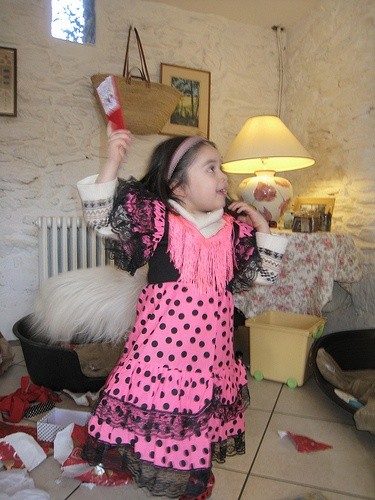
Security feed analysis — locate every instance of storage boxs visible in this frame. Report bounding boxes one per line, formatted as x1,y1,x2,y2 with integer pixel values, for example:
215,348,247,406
4,397,54,418
245,311,327,389
37,407,95,443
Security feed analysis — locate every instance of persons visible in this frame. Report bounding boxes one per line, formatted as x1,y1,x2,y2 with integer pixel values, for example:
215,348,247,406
75,118,288,500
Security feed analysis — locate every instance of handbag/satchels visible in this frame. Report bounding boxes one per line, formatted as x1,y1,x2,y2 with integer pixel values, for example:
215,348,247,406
90,26,182,134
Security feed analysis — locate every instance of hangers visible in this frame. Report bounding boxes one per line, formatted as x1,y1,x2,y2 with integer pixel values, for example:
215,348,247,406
33,216,115,286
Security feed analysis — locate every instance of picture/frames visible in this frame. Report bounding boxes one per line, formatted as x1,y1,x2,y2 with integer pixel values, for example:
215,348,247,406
160,62,211,140
0,46,17,117
294,197,336,217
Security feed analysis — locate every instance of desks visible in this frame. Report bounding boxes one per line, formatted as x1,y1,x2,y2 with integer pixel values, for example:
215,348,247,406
232,228,369,319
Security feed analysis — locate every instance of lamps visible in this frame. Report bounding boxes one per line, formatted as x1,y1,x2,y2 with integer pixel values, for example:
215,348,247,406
220,115,315,225
